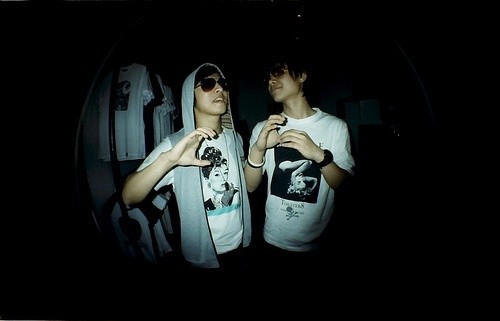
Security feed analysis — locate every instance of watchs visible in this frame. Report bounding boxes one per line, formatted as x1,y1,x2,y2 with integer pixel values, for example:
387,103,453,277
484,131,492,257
315,148,334,168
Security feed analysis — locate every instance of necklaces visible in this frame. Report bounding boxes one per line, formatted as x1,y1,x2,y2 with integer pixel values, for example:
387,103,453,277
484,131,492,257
203,127,235,205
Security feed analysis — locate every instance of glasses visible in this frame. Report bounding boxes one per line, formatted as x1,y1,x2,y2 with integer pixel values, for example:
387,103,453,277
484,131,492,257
261,65,289,82
194,78,230,92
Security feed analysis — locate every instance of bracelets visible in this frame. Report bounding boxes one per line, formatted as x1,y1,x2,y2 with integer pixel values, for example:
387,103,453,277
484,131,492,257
247,155,266,168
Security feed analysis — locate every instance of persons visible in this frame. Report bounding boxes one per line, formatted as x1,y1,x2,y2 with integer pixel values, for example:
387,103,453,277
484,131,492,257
201,145,241,210
122,62,252,268
243,57,355,252
